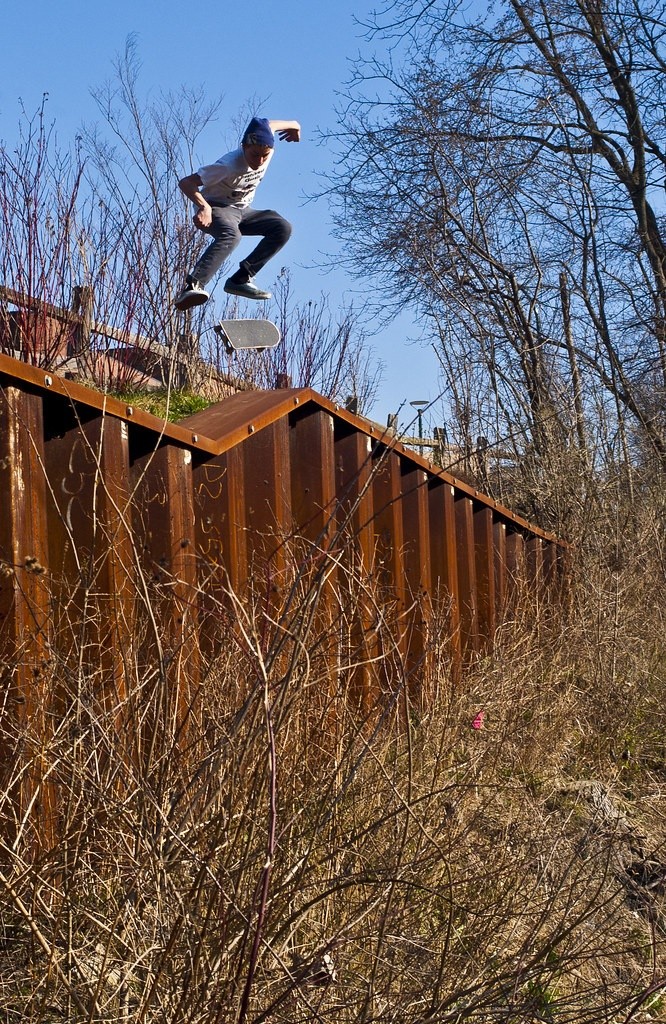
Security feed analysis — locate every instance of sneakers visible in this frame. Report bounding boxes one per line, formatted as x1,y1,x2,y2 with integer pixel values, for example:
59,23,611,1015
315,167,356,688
175,281,209,311
224,275,271,298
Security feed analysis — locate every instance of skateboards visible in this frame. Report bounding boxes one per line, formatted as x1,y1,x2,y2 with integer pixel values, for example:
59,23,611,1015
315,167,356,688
213,318,282,354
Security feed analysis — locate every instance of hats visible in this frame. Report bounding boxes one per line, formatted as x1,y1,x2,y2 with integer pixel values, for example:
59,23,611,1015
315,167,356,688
242,117,274,148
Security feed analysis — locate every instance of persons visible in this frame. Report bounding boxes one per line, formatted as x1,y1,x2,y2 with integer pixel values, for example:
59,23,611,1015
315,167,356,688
174,117,300,311
